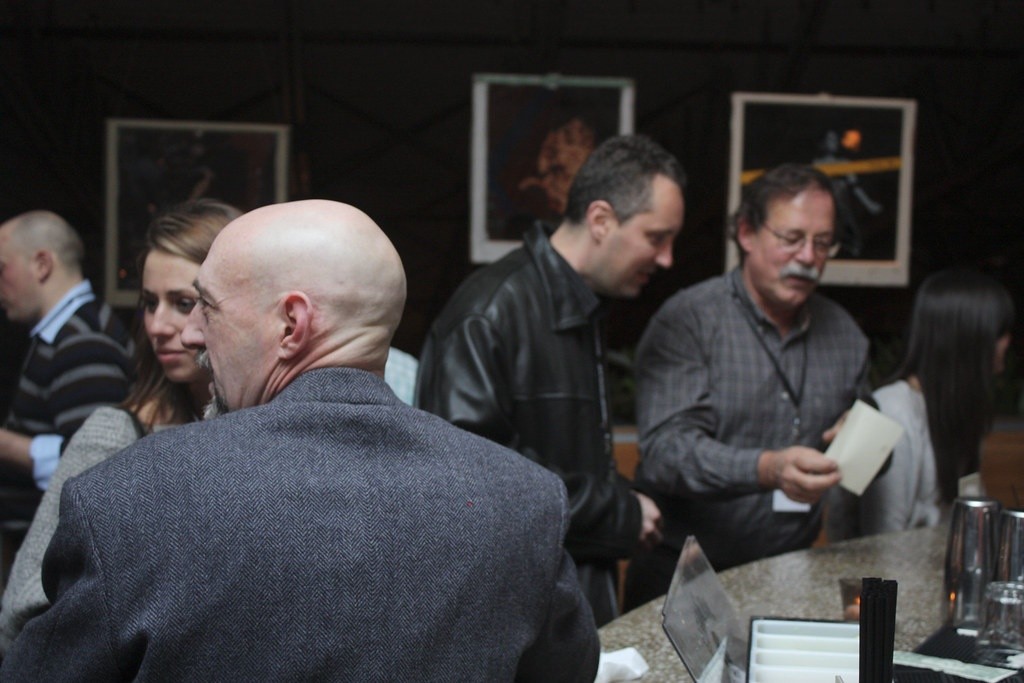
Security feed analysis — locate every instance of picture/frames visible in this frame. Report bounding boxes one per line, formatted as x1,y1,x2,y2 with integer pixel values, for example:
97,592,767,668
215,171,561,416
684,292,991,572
106,120,290,306
726,90,915,286
472,75,637,263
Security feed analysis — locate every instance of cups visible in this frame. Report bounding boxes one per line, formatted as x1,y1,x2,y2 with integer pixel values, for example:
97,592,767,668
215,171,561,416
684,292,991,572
940,498,1023,653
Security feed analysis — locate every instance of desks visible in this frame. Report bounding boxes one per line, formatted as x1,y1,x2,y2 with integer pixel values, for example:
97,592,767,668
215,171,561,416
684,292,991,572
595,518,957,683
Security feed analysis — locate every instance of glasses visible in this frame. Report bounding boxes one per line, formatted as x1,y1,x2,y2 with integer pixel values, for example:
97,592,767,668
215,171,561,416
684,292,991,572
761,222,832,257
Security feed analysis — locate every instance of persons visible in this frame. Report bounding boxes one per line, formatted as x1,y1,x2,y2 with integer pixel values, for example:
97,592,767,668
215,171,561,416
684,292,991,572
1,201,250,623
824,258,1016,545
621,162,892,615
809,132,885,259
415,134,686,629
1,200,601,683
1,209,139,602
